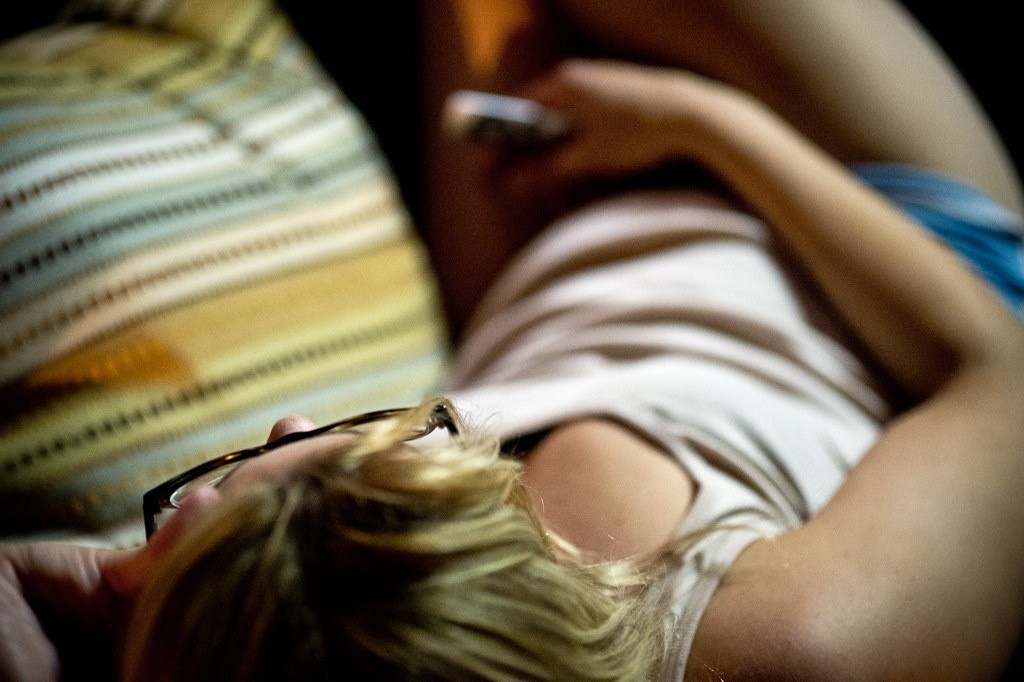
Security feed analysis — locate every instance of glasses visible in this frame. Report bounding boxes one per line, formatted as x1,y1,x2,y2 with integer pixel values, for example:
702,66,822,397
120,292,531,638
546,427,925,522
141,399,466,544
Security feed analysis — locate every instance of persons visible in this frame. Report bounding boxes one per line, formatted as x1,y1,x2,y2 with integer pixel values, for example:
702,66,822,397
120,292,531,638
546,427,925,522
101,1,1024,681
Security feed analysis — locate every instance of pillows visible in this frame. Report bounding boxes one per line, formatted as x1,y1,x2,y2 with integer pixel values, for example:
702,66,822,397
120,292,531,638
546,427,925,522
0,0,454,541
